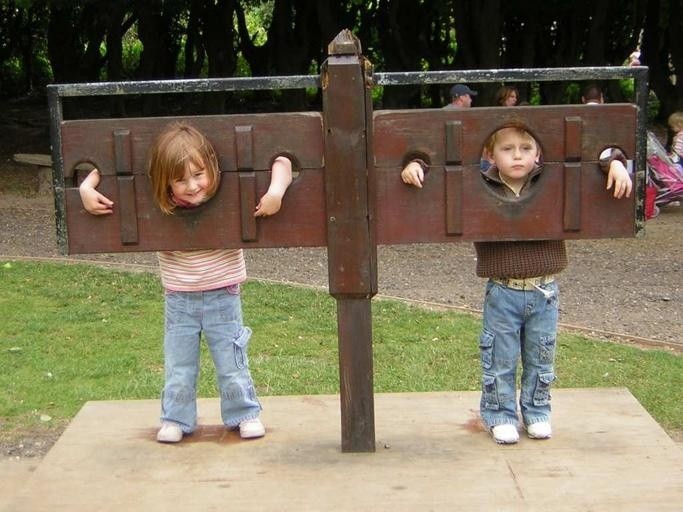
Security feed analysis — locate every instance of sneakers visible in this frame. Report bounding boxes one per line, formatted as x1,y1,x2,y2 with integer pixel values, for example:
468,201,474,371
527,423,552,438
156,424,184,442
240,416,265,438
493,424,518,444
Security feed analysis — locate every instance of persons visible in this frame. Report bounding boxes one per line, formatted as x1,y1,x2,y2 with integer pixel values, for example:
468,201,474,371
581,84,605,106
400,125,633,444
666,110,683,164
79,119,292,443
440,85,478,109
495,86,519,108
644,174,660,222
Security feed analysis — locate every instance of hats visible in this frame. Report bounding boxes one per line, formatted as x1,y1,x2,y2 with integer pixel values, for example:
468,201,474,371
450,85,477,96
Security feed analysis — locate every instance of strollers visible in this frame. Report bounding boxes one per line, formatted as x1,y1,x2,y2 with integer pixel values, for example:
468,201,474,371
643,130,683,219
